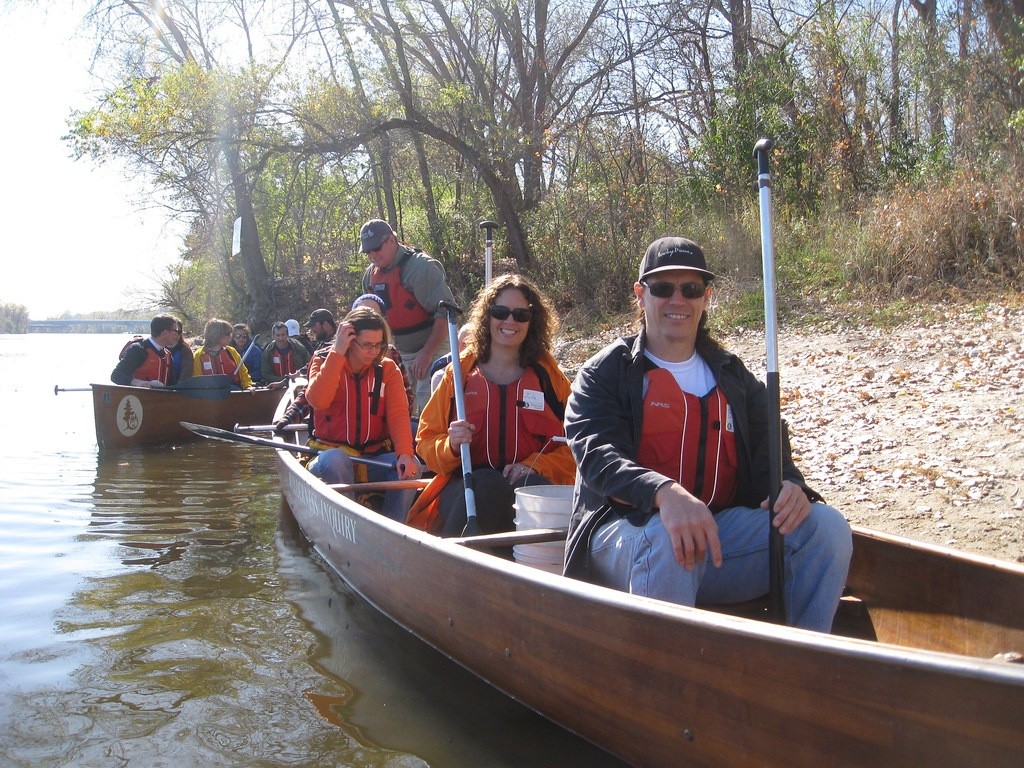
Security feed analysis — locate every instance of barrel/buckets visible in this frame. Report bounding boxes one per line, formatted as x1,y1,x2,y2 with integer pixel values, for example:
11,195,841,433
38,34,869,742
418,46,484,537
513,436,574,575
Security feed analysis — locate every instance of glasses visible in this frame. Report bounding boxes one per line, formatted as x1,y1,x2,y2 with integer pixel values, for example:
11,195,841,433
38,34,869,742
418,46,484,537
163,328,182,336
353,338,386,350
491,302,533,322
363,238,389,254
641,280,708,299
235,334,247,339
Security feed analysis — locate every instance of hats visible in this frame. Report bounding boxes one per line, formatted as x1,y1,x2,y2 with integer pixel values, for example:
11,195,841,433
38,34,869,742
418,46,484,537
352,294,387,317
358,219,392,255
638,236,715,284
303,309,334,327
285,319,300,337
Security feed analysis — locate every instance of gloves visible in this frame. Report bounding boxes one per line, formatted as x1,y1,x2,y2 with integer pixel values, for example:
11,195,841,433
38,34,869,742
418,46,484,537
273,417,292,437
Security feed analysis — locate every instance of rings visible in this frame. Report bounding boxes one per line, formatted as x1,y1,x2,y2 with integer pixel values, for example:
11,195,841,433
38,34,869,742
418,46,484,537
412,471,416,476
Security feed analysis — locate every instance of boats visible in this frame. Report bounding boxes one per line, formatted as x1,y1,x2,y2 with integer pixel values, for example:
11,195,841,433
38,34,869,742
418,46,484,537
268,370,1023,768
88,380,288,449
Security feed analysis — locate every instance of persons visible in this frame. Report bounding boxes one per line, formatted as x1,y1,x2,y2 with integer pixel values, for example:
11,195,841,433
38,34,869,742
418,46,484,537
166,318,194,385
262,322,311,385
565,236,852,635
285,318,314,356
273,294,421,524
267,309,337,392
193,318,256,396
358,219,456,418
110,313,178,388
230,324,262,382
407,275,577,537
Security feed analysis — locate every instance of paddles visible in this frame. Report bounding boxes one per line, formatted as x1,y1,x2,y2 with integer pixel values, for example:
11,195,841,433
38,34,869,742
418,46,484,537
177,419,404,473
436,299,493,556
479,221,500,286
55,373,232,401
233,333,261,377
751,139,786,625
233,421,308,434
